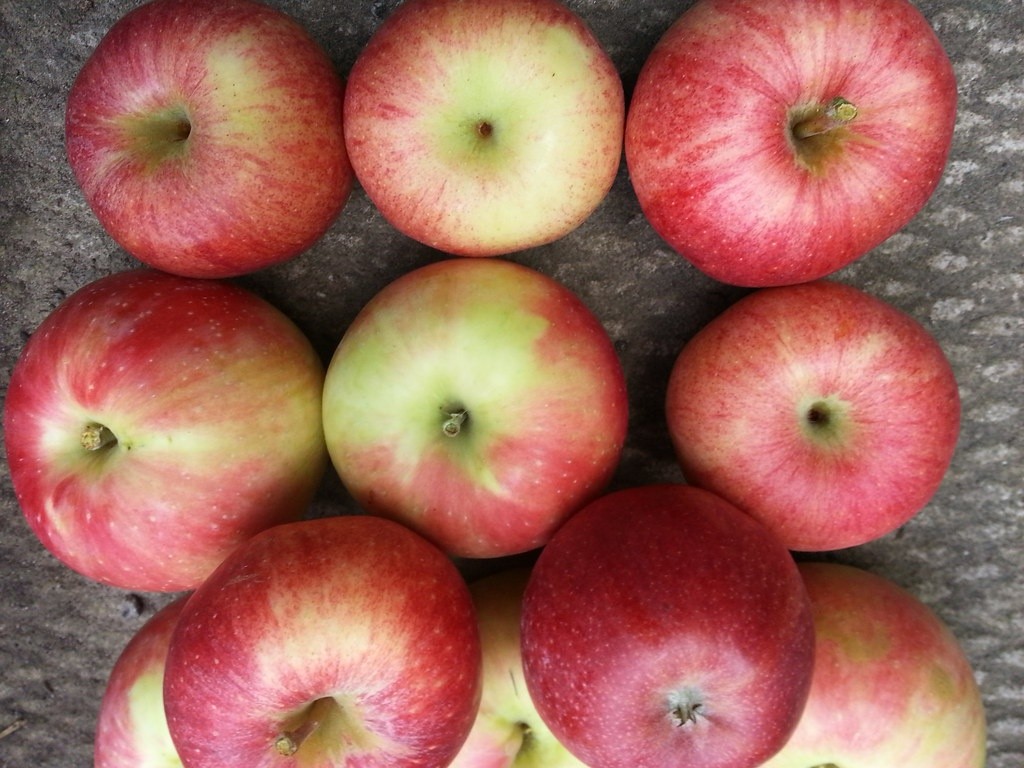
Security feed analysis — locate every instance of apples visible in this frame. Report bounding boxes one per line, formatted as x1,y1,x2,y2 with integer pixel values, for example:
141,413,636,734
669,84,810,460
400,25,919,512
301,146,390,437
164,514,483,768
5,267,327,595
322,257,631,558
663,279,961,553
439,551,590,768
620,1,956,288
65,0,355,279
520,485,817,768
93,595,190,767
342,0,624,257
753,559,987,768
